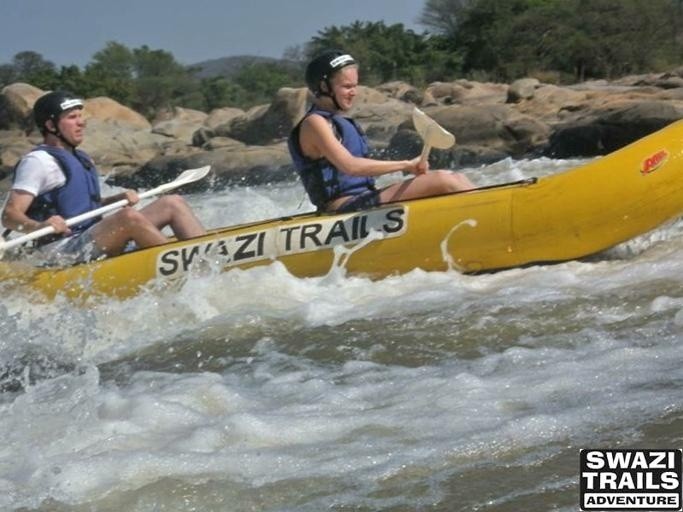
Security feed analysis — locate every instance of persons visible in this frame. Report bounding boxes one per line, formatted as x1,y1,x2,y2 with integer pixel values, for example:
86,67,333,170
1,90,207,266
287,51,477,211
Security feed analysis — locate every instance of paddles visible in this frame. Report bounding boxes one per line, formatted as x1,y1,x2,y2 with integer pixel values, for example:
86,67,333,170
412,107,456,162
0,164,212,251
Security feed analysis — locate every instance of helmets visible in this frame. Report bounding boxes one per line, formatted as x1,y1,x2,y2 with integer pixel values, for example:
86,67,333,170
305,50,356,91
34,92,84,133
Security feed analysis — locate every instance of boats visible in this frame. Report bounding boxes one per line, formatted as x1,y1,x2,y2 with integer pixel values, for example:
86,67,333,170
0,117,683,317
